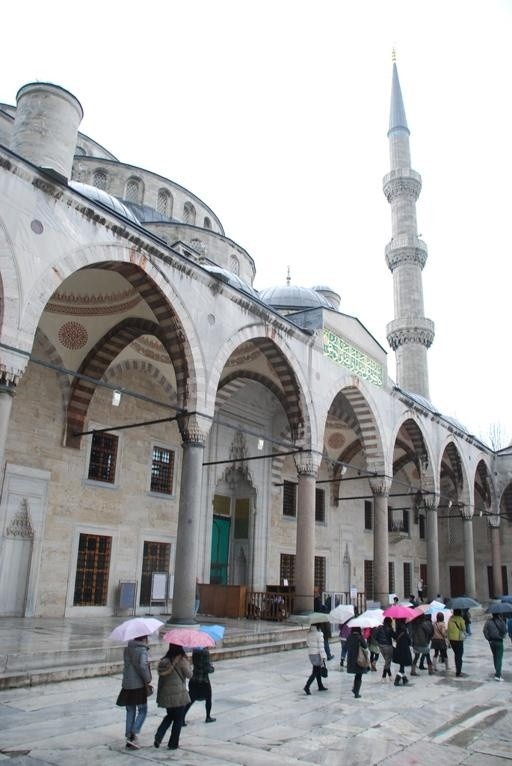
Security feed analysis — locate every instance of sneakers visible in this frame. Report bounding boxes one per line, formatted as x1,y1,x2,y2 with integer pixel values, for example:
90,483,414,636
125,736,139,749
495,673,505,681
327,654,334,659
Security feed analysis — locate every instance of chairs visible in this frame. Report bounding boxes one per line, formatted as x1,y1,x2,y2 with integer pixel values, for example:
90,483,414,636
259,598,288,622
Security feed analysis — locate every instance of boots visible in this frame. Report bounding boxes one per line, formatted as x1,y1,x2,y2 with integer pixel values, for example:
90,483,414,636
338,657,463,686
318,684,328,690
304,685,311,694
352,680,362,697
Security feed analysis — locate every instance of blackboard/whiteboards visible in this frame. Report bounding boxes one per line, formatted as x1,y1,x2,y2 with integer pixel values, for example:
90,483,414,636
120,581,138,609
150,572,169,602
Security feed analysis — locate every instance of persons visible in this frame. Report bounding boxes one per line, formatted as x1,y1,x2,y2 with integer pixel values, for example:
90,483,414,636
182,646,216,726
299,579,512,698
154,643,194,749
115,635,153,750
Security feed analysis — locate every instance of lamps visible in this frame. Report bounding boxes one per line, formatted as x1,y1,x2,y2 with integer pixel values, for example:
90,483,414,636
257,436,265,451
448,499,452,509
479,510,483,518
340,464,346,475
111,389,121,407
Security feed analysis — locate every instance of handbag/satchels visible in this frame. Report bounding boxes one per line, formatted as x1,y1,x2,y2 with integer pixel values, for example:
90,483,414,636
357,641,370,668
320,661,327,677
459,629,468,641
444,638,452,647
143,683,154,696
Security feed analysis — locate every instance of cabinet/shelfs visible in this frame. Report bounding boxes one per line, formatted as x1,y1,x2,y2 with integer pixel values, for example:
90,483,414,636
196,582,248,622
264,585,320,613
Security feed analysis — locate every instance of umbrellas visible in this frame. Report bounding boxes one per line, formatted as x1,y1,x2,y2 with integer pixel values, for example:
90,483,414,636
107,617,225,668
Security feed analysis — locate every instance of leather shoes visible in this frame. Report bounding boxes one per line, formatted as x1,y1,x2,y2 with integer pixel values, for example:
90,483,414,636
154,740,160,748
182,722,187,726
168,745,178,749
206,717,216,722
